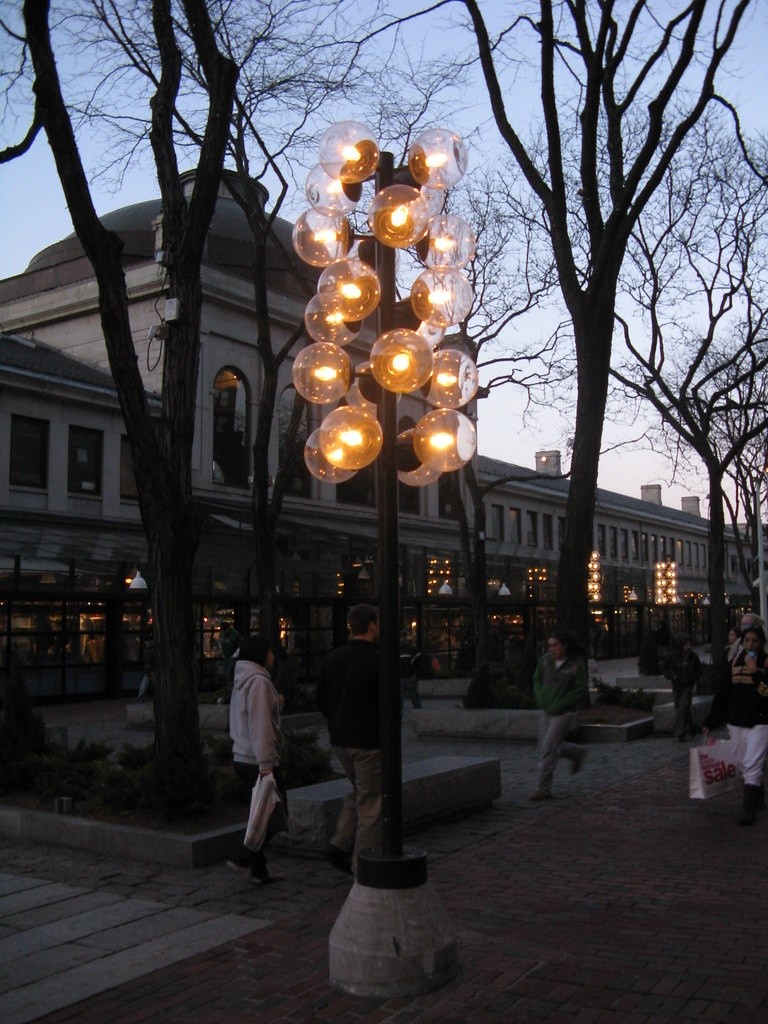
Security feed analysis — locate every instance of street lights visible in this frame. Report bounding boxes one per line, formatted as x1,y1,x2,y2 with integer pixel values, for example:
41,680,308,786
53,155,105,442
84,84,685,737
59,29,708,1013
289,121,481,892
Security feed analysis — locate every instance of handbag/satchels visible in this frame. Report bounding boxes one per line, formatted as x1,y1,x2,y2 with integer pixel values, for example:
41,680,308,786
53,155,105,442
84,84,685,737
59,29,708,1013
689,734,739,799
244,772,281,852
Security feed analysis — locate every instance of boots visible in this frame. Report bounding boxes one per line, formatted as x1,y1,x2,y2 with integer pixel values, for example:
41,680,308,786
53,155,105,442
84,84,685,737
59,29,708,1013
737,777,766,825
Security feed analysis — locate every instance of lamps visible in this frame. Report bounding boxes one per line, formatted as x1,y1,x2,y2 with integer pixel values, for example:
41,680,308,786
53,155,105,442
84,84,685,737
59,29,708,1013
364,549,373,565
498,580,510,596
629,587,638,600
356,565,370,580
352,552,361,569
118,551,147,589
438,573,453,596
703,596,710,605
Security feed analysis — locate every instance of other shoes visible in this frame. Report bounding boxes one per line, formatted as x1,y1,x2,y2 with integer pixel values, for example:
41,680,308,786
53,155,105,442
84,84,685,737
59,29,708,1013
571,746,587,775
321,843,352,869
529,787,553,800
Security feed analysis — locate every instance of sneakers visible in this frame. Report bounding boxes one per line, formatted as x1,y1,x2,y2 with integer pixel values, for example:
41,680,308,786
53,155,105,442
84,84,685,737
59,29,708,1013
224,848,286,884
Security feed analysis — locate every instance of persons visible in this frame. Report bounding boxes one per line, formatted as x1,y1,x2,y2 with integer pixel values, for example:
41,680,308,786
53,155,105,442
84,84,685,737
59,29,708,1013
227,635,285,884
402,646,424,710
668,636,703,733
219,621,243,655
701,613,768,827
527,632,587,801
314,603,382,883
